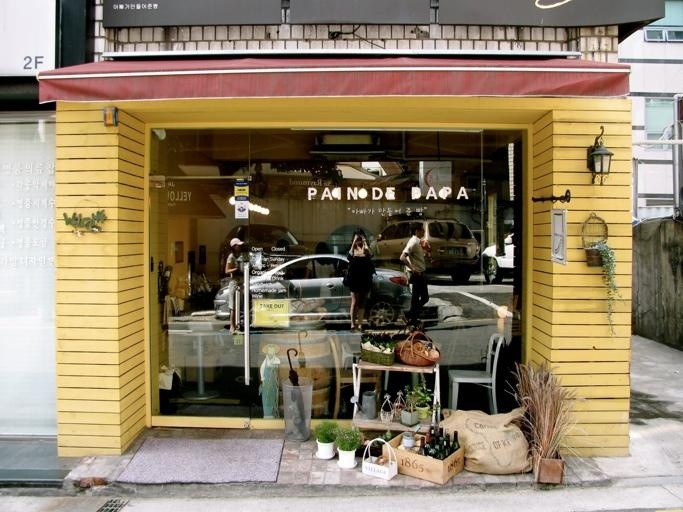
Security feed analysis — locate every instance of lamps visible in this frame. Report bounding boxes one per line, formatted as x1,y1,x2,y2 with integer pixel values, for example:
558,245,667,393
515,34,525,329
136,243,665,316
586,125,614,187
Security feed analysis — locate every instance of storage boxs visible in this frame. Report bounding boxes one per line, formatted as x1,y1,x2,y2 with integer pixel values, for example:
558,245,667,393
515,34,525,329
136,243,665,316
381,431,465,485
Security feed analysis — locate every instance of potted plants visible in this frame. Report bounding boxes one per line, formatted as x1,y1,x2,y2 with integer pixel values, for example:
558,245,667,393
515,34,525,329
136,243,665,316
400,377,431,427
584,239,627,337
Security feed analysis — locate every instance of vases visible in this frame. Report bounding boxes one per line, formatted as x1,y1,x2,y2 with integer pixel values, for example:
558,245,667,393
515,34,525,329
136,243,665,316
314,437,358,469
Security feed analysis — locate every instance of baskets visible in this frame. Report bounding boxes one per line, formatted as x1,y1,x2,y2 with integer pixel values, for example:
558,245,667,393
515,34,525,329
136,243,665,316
362,438,397,480
397,332,442,366
361,341,397,366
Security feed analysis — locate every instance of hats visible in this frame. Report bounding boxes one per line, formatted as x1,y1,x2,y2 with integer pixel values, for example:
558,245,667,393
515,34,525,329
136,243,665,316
230,238,244,247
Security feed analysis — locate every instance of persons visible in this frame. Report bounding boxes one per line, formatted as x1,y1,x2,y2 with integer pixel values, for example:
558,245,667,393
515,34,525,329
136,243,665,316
276,237,294,280
342,229,375,331
398,223,431,321
304,240,336,279
225,238,245,334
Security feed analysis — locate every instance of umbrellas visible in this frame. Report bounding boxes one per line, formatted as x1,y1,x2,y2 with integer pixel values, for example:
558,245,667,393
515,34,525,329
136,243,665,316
296,328,310,368
286,348,309,441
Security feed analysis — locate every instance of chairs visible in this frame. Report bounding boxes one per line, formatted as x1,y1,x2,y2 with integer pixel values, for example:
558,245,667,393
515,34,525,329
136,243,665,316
325,332,504,420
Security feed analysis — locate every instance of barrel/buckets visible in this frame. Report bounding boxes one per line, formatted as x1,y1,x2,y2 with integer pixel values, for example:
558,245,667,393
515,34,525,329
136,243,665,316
284,377,312,441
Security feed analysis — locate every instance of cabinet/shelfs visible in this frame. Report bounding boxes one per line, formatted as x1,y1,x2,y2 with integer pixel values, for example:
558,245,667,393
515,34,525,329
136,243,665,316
351,359,441,434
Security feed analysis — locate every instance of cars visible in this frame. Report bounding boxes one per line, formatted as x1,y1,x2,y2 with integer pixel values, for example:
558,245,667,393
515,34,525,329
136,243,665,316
219,224,314,277
213,254,408,329
481,231,513,280
260,291,512,388
368,216,479,283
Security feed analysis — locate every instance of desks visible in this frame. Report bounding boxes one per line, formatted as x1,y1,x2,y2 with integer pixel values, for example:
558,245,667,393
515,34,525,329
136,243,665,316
167,329,230,401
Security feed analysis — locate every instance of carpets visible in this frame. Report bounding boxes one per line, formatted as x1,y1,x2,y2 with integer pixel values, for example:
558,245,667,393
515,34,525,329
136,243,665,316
115,435,285,485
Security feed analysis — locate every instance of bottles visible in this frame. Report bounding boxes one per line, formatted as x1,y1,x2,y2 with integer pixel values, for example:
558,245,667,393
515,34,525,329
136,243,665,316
416,424,461,461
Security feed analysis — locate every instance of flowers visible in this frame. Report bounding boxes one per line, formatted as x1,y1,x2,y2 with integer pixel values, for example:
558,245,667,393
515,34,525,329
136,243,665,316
313,420,361,451
